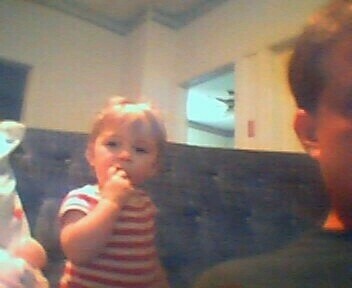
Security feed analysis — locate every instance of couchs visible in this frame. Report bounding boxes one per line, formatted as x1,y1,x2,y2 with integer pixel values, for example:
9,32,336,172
10,128,331,288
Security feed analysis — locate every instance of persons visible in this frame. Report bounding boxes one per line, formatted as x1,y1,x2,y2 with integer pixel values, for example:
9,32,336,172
0,119,53,287
191,0,352,286
58,92,172,286
